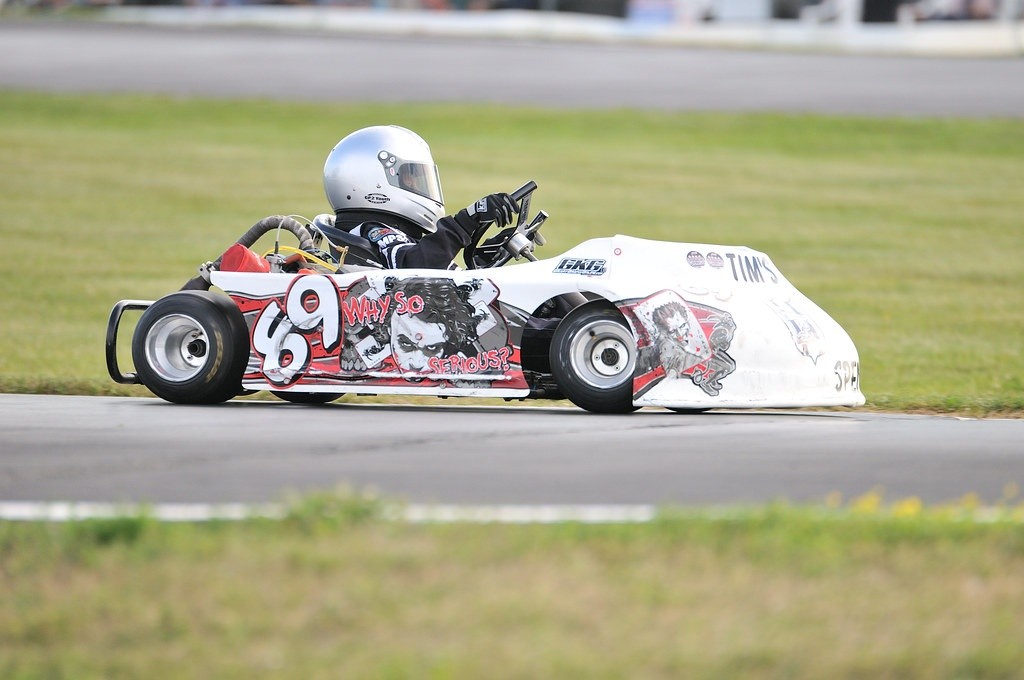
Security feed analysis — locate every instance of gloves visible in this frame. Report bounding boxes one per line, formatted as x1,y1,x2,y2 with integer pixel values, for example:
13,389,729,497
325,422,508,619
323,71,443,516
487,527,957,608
455,192,520,236
479,225,547,265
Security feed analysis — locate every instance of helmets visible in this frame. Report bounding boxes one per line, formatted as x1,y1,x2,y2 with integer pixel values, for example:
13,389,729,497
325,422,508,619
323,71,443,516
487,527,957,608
323,124,445,234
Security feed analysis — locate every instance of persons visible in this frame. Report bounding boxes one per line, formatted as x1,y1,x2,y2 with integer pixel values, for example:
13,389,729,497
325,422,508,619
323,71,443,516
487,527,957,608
308,123,521,269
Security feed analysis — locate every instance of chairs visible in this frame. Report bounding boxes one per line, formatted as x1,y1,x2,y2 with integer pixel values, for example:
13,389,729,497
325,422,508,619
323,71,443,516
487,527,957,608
313,214,382,268
